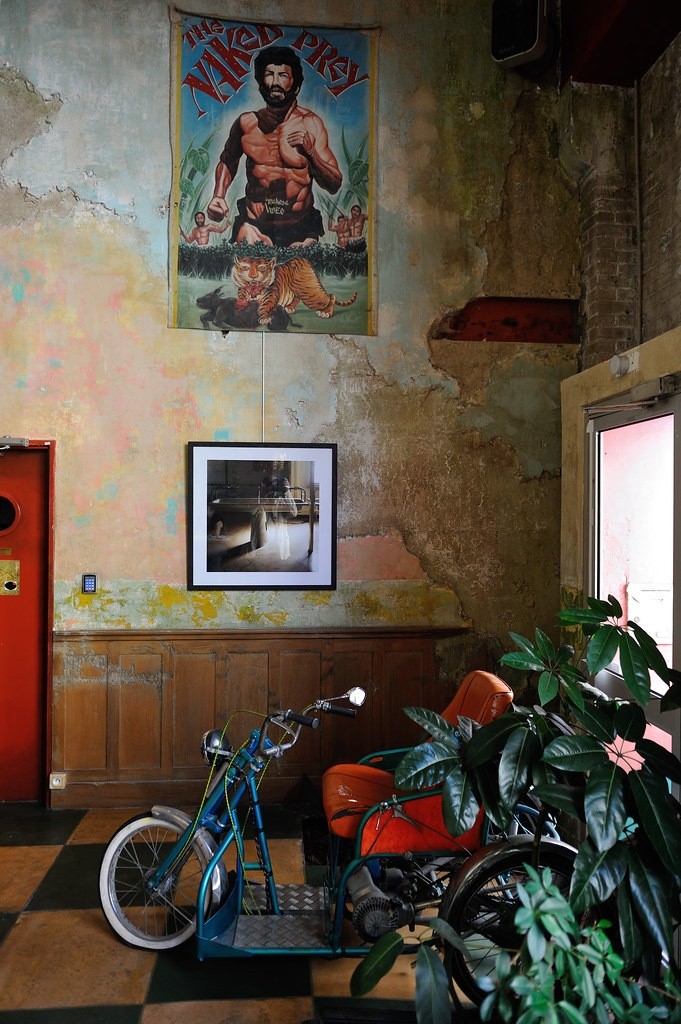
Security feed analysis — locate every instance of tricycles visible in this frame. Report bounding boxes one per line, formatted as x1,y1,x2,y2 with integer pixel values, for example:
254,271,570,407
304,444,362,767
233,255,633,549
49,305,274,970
99,669,626,1018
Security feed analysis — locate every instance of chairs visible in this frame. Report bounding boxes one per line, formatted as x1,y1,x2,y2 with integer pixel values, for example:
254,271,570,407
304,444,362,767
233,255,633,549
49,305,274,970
322,671,514,855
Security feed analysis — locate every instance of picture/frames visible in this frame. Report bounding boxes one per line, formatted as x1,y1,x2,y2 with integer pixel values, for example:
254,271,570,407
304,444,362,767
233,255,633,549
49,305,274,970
186,441,338,591
